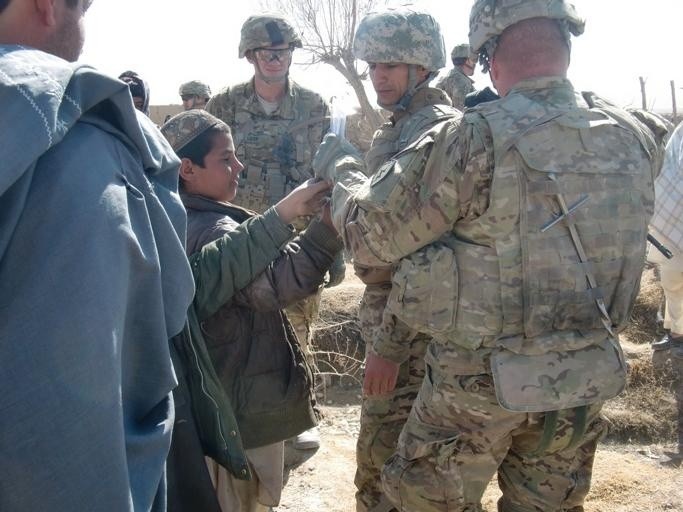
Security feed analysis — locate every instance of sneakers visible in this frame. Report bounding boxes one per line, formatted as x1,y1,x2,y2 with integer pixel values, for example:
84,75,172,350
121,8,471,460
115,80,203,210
292,427,319,452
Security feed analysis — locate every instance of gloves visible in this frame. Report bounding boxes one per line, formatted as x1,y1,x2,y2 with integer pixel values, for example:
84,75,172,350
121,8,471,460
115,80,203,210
310,132,360,182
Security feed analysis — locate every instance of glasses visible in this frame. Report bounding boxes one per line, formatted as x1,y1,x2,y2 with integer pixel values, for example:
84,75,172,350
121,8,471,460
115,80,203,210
252,46,295,60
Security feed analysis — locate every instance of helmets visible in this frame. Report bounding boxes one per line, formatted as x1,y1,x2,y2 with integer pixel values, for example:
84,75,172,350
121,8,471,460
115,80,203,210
352,8,445,72
180,81,211,98
238,16,302,59
451,44,476,60
468,0,586,52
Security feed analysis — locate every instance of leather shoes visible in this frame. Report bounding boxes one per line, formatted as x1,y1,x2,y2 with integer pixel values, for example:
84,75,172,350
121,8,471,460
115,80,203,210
651,331,681,351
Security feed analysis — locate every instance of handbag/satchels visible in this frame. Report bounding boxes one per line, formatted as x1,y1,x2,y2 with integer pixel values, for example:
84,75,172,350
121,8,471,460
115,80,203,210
490,336,626,413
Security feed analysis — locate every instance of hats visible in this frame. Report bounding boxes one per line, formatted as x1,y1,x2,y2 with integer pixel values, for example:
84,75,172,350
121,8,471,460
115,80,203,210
161,109,219,153
118,75,145,98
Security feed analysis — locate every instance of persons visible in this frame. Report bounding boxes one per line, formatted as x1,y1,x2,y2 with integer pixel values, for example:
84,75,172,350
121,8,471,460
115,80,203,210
1,0,197,512
313,1,669,512
163,178,333,512
647,117,683,351
117,70,151,118
353,4,465,512
204,15,347,449
158,108,341,512
180,81,214,111
436,43,477,113
